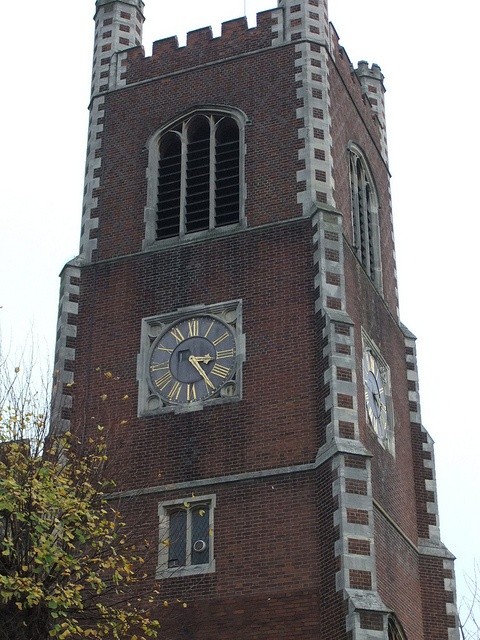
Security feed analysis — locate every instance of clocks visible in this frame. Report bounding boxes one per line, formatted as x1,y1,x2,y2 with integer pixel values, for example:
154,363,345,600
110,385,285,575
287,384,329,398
146,313,237,405
361,346,390,440
